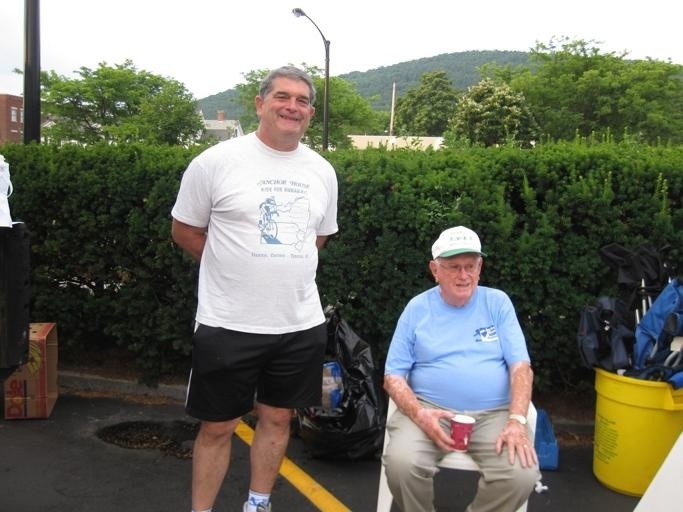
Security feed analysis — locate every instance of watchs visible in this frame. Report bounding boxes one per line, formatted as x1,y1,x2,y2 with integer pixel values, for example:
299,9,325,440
508,413,526,425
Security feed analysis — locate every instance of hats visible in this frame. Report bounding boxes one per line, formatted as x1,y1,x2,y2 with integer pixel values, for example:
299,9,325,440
430,224,489,259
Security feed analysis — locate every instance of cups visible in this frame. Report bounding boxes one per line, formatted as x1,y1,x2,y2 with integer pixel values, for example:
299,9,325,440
449,415,476,452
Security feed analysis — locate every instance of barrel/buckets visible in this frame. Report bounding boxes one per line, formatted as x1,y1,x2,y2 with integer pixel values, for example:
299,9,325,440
590,367,683,497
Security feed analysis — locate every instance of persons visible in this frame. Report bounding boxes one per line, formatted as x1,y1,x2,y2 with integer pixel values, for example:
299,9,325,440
167,64,342,510
381,224,539,512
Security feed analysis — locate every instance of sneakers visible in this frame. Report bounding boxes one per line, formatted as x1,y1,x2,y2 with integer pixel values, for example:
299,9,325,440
242,502,272,512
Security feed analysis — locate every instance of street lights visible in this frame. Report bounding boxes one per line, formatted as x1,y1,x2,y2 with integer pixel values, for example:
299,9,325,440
293,7,330,153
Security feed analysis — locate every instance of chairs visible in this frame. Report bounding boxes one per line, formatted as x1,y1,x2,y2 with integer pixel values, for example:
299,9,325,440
379,387,542,512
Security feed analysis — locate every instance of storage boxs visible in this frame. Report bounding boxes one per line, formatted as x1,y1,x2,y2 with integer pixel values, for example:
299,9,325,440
4,321,62,422
